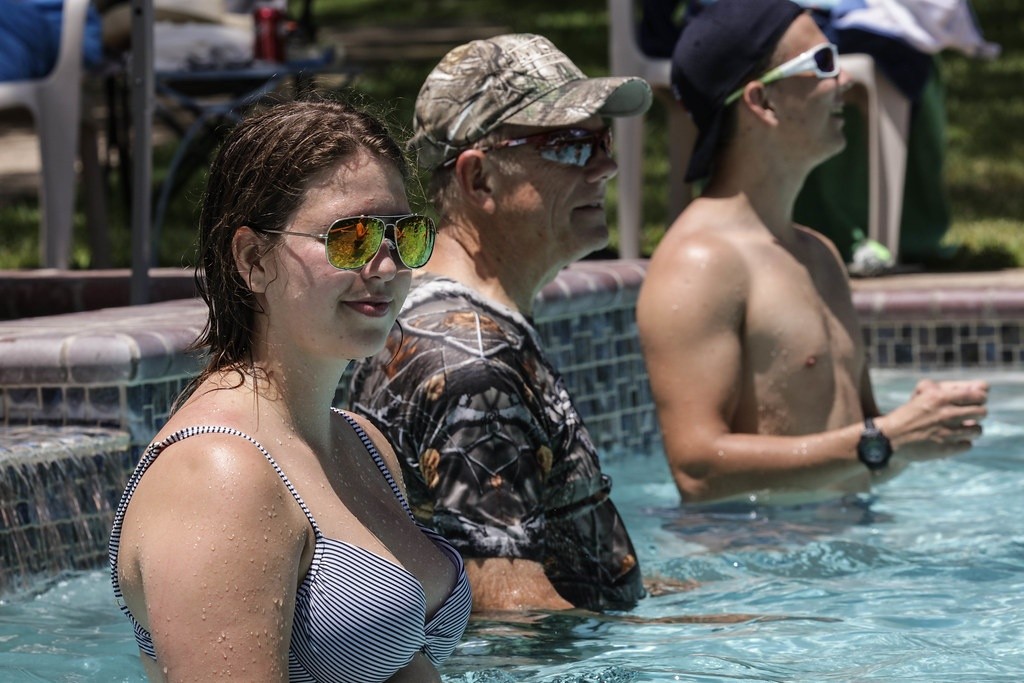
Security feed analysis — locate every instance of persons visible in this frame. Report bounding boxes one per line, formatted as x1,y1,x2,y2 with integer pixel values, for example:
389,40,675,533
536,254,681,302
108,93,475,682
346,28,846,660
634,0,993,576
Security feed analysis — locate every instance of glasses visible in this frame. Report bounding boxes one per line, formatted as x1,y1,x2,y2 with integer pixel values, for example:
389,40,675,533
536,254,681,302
444,119,615,167
261,214,439,271
725,42,839,106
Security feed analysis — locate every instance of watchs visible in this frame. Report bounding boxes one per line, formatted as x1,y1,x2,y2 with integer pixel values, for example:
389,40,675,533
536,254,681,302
853,411,894,479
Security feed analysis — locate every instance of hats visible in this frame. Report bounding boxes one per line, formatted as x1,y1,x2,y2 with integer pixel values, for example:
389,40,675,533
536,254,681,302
672,0,806,181
407,33,652,172
